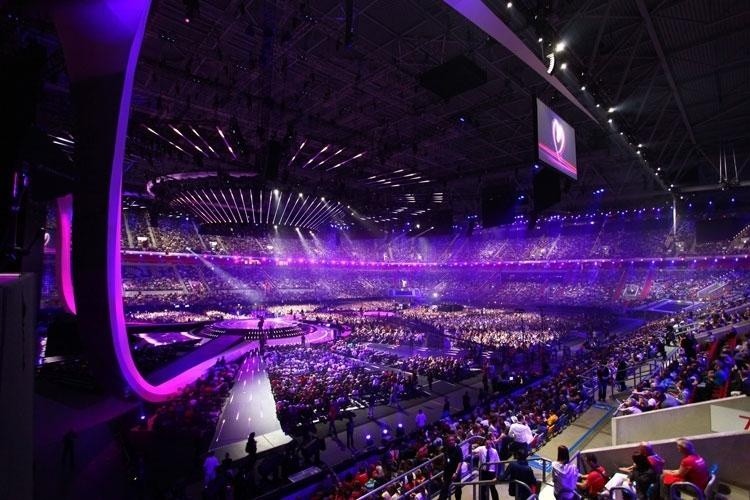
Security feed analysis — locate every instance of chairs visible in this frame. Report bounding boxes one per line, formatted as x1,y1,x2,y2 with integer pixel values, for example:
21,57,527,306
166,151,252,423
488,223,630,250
685,333,738,403
670,474,719,500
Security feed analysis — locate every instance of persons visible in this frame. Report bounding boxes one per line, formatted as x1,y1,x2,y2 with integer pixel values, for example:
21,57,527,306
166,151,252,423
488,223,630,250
44,207,750,500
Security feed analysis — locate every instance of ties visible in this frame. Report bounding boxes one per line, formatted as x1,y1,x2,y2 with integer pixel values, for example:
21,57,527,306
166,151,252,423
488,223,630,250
486,449,490,472
